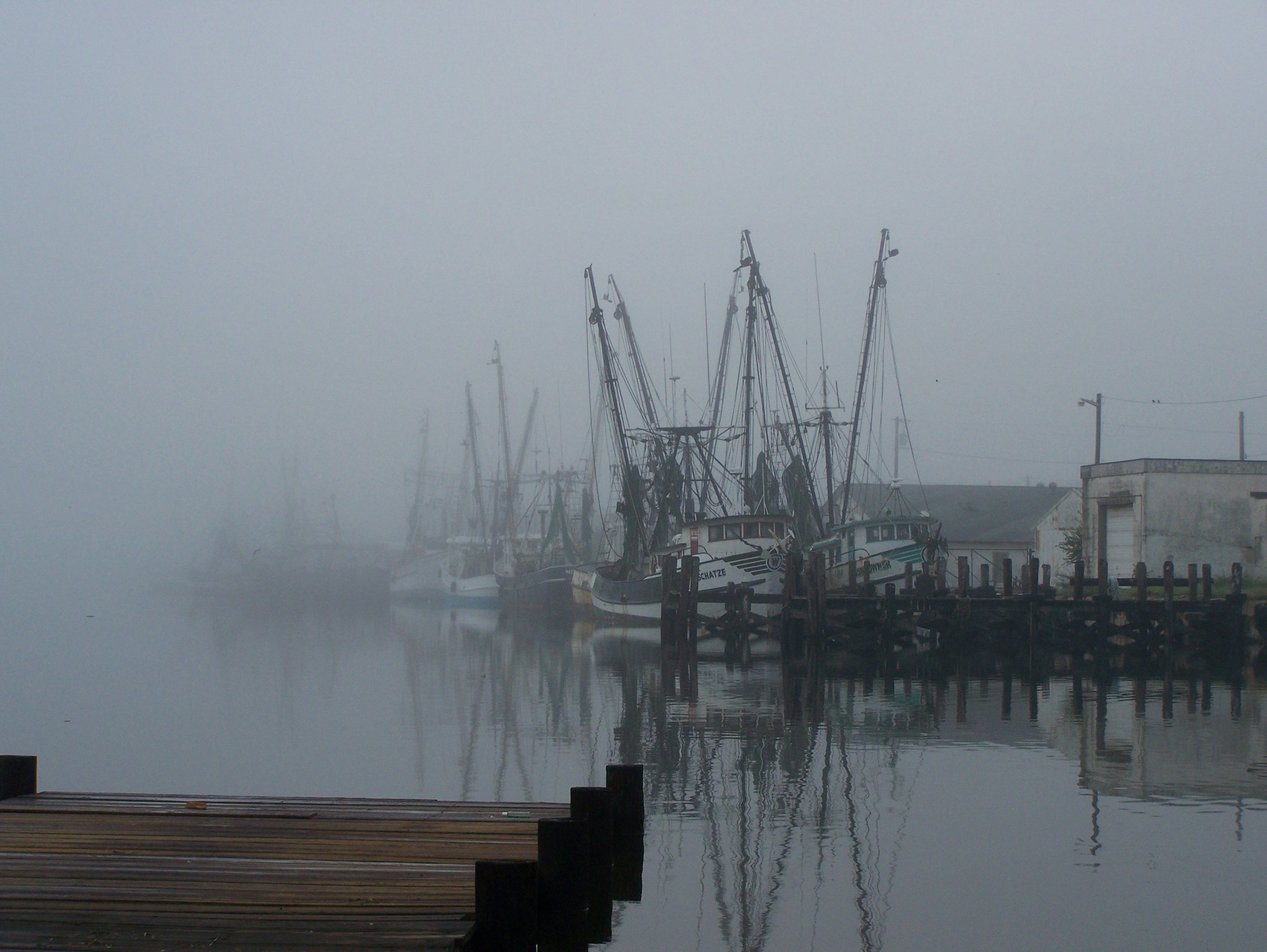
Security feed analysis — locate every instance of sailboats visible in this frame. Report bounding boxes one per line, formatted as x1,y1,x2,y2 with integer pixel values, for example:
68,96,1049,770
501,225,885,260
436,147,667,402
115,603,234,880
378,224,951,677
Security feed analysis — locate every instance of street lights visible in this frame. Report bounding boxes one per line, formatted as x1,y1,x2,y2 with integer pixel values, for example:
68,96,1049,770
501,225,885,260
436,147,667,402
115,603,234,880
1076,390,1102,465
